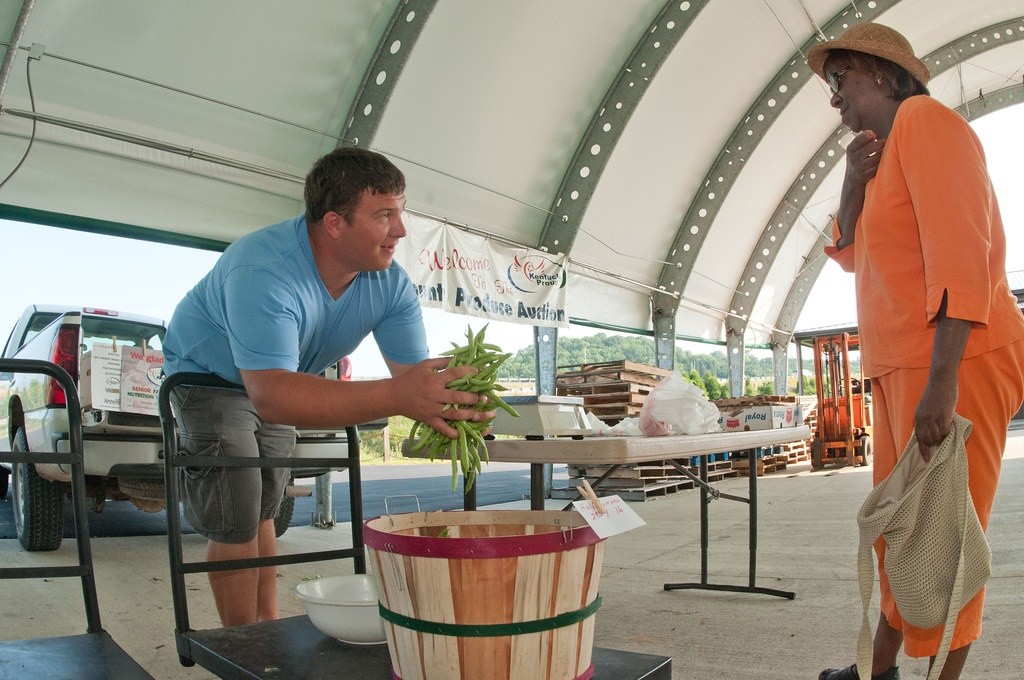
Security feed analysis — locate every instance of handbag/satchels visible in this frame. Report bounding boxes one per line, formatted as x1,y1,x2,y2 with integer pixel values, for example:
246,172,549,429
856,412,993,680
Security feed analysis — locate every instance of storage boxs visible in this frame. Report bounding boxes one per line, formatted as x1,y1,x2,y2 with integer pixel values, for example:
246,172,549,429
719,404,803,431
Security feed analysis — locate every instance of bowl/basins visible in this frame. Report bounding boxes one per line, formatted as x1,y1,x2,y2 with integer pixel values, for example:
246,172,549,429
295,574,387,645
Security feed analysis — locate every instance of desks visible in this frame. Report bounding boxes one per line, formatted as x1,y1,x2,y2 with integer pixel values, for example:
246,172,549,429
403,426,812,601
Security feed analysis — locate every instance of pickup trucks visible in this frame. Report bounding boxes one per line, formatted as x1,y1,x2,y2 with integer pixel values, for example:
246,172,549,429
0,303,354,553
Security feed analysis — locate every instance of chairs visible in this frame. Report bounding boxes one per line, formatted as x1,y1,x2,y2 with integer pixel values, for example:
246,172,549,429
857,379,871,397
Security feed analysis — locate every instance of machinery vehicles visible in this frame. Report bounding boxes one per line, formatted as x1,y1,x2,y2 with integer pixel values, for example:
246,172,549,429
810,331,876,470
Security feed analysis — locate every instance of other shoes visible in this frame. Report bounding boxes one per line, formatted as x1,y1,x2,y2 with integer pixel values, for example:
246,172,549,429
818,665,900,680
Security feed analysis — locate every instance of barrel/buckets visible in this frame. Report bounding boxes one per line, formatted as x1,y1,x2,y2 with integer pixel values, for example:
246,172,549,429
363,495,607,680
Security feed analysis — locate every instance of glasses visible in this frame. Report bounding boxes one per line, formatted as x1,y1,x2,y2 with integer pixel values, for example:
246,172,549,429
826,66,853,93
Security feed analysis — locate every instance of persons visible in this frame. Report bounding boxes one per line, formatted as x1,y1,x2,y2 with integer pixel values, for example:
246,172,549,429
808,22,1024,680
162,148,496,629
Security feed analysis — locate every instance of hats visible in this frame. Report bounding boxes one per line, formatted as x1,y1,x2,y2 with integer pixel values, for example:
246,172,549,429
807,22,931,86
